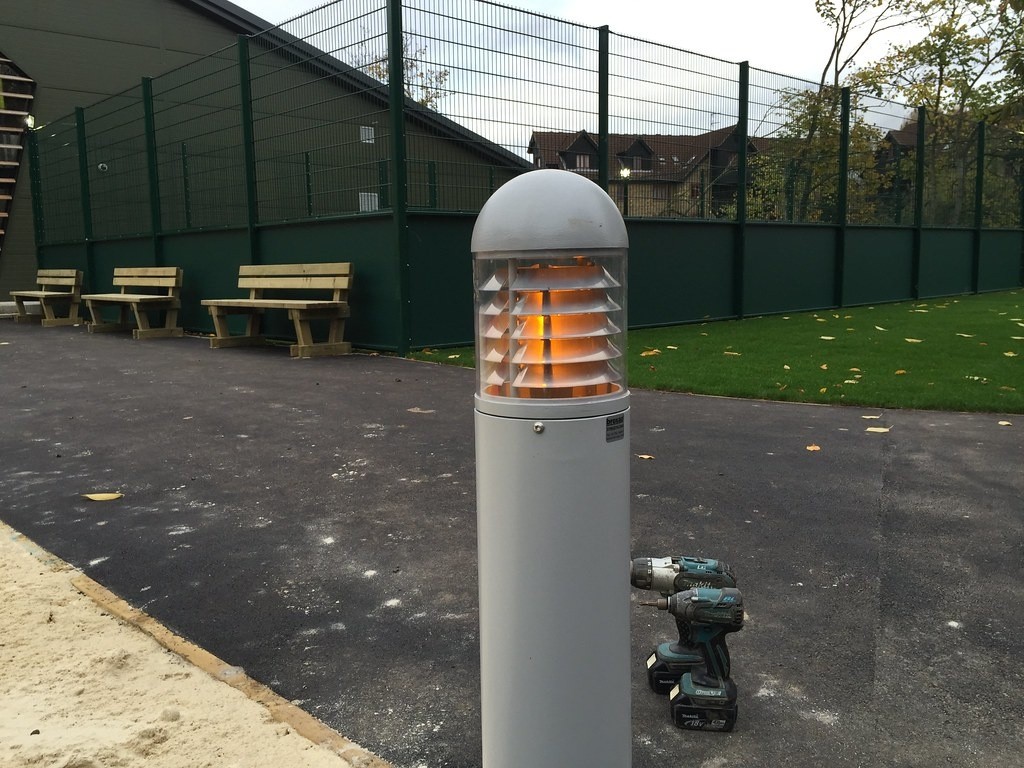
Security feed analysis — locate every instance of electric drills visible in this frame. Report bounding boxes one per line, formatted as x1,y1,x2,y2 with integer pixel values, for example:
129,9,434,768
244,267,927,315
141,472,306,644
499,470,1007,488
638,587,743,733
630,556,736,694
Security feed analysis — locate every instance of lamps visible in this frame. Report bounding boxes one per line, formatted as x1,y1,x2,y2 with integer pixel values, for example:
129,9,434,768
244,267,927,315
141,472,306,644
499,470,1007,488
23,114,47,133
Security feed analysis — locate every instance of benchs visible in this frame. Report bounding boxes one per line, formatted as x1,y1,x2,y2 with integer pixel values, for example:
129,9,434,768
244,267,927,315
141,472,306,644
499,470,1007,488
9,269,83,327
80,266,183,339
200,262,354,357
